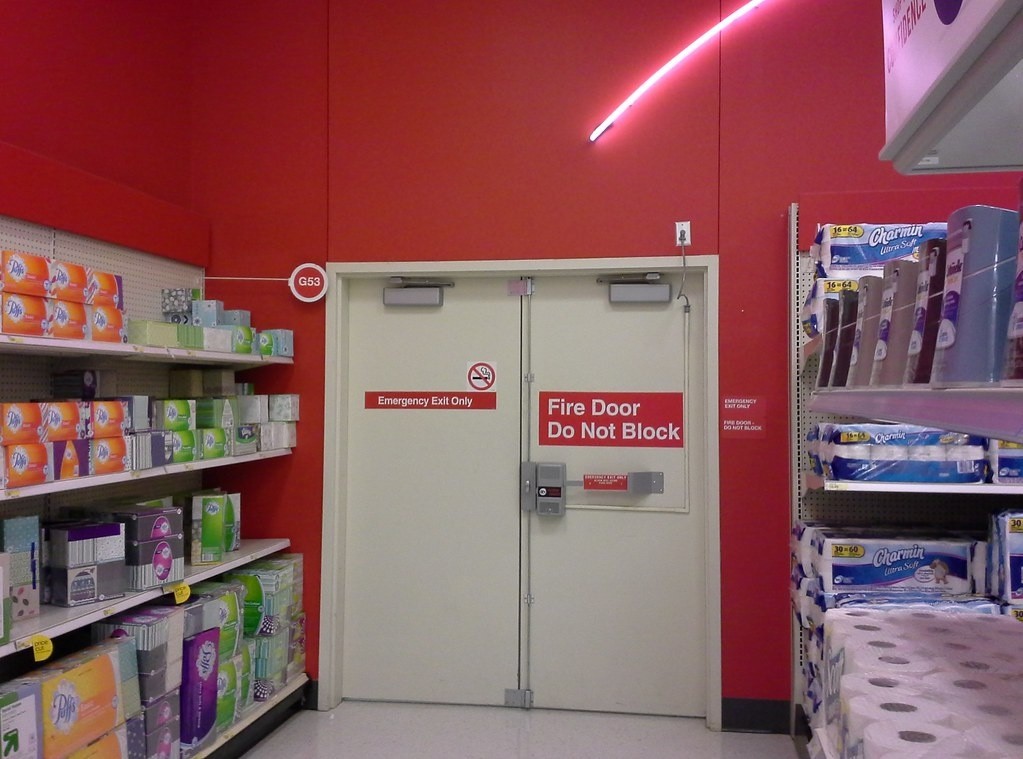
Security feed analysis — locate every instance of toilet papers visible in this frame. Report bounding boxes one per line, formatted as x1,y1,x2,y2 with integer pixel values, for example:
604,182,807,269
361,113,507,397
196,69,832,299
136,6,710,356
806,422,1023,485
800,222,948,341
794,508,1023,759
